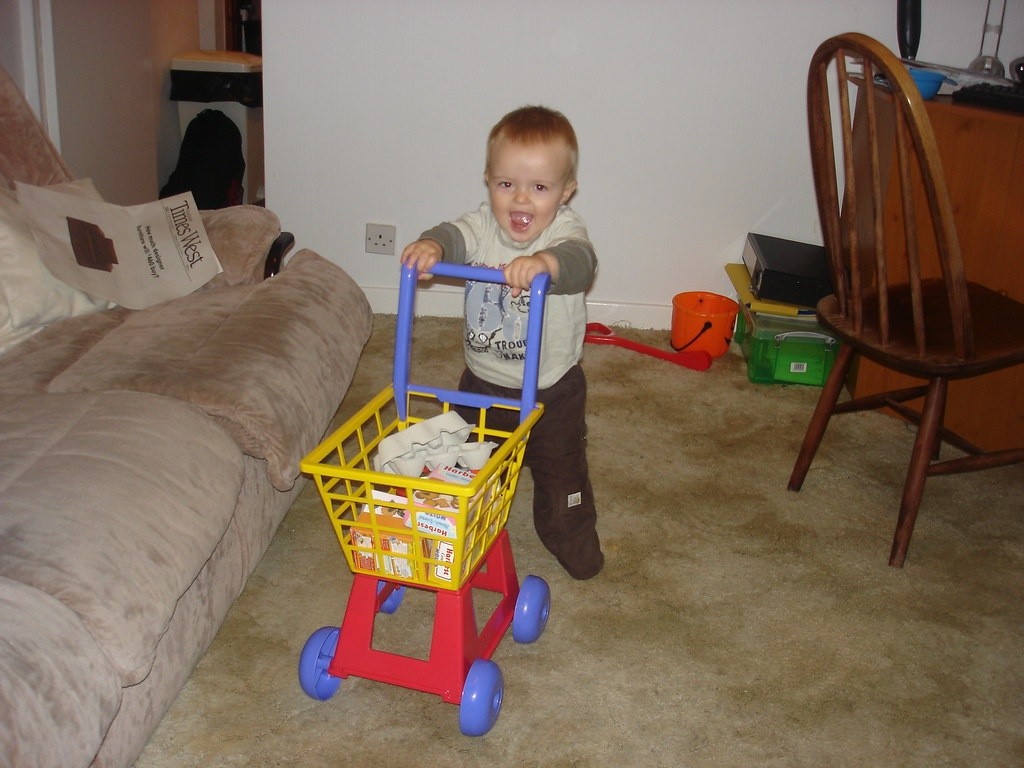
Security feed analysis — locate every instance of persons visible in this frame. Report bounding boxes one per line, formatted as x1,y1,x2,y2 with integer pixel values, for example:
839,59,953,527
401,107,604,579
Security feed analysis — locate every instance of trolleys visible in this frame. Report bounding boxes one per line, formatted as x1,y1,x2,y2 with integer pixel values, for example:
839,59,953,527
298,257,551,737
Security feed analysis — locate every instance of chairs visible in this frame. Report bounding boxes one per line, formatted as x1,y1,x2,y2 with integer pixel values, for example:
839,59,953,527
786,30,1023,565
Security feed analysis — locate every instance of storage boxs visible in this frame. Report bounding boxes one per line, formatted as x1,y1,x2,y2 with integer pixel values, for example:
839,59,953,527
734,300,835,387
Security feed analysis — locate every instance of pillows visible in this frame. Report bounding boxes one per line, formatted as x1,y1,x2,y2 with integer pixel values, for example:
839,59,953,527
0,176,114,352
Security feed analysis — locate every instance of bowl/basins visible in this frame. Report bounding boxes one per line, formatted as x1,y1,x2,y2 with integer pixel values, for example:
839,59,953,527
906,69,946,99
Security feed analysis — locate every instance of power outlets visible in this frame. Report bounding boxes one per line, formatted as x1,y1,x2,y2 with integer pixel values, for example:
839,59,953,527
366,223,396,256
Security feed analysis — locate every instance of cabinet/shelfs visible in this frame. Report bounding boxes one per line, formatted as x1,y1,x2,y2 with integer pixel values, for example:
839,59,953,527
838,71,1023,458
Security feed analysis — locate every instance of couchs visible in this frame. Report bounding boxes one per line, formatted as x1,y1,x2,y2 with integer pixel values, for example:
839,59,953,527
0,68,371,767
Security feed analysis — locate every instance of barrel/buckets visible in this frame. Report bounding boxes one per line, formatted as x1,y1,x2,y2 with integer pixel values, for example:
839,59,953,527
669,291,740,360
